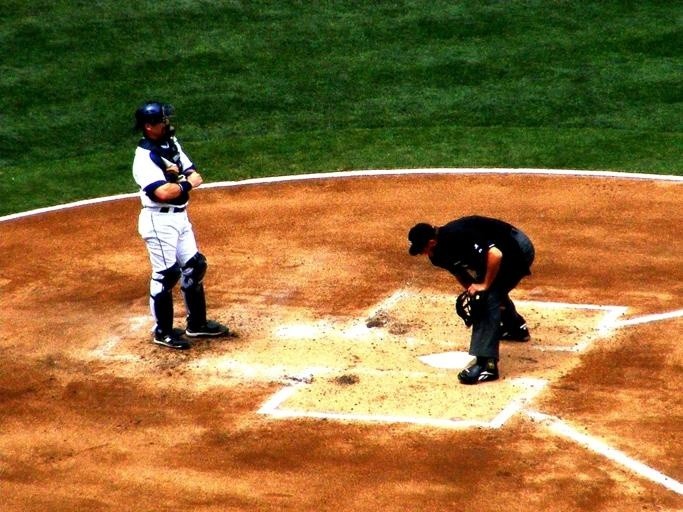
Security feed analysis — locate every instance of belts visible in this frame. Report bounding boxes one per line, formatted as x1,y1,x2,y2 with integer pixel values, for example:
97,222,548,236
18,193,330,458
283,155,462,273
160,206,187,212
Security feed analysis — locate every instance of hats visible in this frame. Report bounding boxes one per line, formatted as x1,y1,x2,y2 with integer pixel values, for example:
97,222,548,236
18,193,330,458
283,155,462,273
408,224,433,256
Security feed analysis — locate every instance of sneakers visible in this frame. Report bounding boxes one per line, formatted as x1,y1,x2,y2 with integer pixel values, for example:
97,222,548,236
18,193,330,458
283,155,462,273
499,323,531,342
185,320,230,338
458,363,498,381
153,327,193,350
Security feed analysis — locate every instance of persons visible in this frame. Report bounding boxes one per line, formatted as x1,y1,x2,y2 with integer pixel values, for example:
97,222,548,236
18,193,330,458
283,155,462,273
405,214,535,383
130,101,228,349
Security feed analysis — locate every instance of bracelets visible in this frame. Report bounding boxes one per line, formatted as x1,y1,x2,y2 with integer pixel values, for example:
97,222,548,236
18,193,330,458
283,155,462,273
179,171,193,179
177,180,192,193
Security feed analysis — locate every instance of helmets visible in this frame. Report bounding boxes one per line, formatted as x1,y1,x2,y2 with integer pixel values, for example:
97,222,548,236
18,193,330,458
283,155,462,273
135,100,177,141
